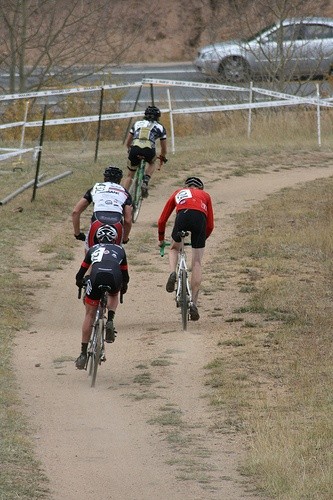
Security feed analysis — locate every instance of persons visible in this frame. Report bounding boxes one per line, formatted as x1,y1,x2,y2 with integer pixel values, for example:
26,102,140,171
76,224,129,369
72,166,132,254
158,176,214,321
123,106,168,198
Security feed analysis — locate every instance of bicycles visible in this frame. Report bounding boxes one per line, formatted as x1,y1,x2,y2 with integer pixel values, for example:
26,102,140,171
160,229,197,331
76,280,125,389
126,147,169,223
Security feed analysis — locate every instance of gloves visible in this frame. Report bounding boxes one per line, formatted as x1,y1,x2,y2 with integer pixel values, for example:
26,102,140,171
74,233,86,242
122,238,129,244
159,155,168,165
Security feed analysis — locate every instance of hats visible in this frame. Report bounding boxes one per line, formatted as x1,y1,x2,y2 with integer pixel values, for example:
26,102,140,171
96,225,118,244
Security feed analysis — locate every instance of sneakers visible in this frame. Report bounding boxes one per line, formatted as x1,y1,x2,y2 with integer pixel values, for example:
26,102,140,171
166,271,177,293
75,353,87,370
141,184,149,199
106,320,115,344
190,304,199,321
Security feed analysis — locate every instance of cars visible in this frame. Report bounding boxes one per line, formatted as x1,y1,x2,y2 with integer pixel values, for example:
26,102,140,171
192,15,332,85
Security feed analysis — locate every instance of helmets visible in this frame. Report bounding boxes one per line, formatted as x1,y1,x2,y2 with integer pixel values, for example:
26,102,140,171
185,177,204,190
145,106,161,117
104,167,124,178
